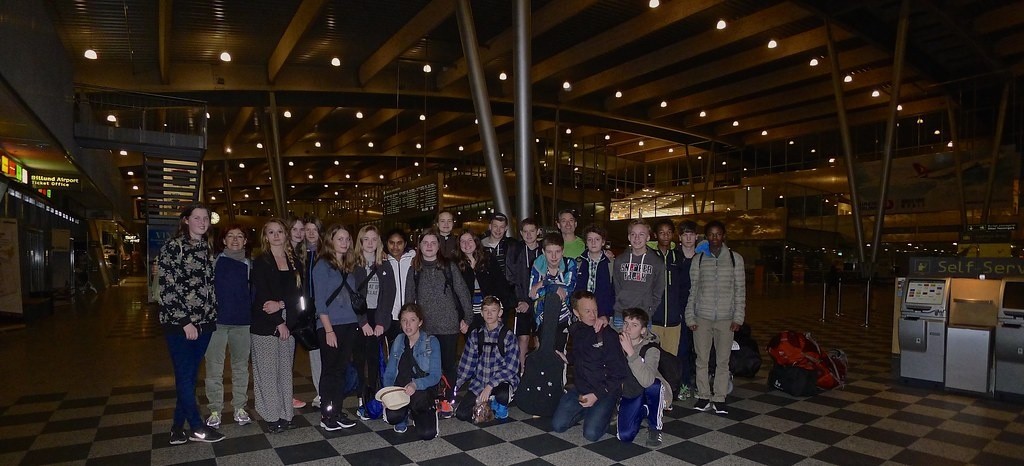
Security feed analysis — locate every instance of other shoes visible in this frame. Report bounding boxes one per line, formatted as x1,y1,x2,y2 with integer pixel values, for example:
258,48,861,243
312,395,321,407
281,419,296,429
646,430,662,448
267,421,284,434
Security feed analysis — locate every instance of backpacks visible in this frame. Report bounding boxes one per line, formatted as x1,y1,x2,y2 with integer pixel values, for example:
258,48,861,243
638,342,684,401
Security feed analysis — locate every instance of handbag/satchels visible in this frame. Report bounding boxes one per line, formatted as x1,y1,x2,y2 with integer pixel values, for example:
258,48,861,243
767,330,850,395
291,310,319,351
351,293,368,315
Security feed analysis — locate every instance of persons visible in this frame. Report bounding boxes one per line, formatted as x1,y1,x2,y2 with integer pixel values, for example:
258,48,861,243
553,291,622,442
685,220,747,413
157,202,226,445
384,303,441,440
152,223,254,426
300,219,322,408
249,218,302,432
353,225,396,420
286,212,306,409
594,308,669,445
386,230,416,360
452,296,520,421
404,229,473,419
434,209,457,257
312,225,357,430
457,210,700,410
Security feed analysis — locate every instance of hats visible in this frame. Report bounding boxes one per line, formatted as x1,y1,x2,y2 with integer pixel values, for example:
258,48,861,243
374,386,410,410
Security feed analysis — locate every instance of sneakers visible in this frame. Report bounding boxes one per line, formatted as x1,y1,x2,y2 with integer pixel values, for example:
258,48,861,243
494,402,509,418
169,427,187,445
394,418,408,432
357,405,371,420
441,400,454,419
712,402,728,415
206,413,221,426
336,413,356,429
293,399,306,409
189,425,226,443
234,409,251,426
694,399,710,411
321,416,342,431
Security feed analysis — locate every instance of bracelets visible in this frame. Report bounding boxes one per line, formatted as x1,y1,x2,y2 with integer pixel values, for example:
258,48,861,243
281,301,285,309
325,331,334,333
